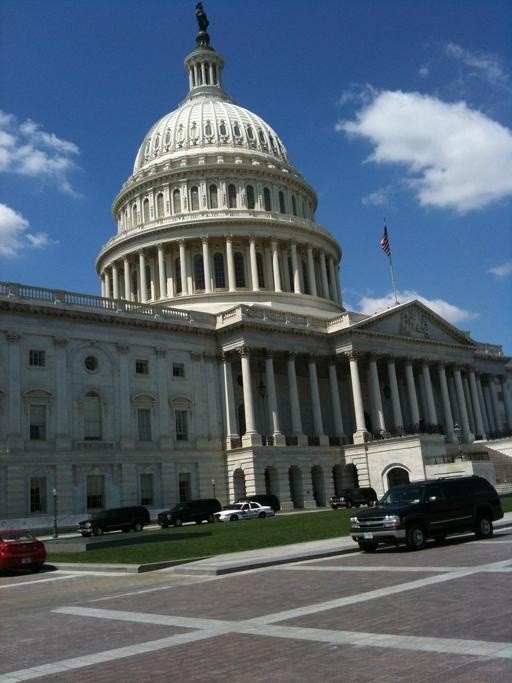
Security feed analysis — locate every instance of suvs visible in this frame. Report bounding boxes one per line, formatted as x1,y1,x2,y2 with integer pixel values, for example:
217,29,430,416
223,494,280,514
158,497,222,528
78,505,151,536
329,487,378,510
347,473,504,552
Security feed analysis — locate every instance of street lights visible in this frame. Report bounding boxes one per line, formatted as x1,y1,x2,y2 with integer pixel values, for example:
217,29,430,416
453,419,464,460
211,477,217,496
51,487,59,538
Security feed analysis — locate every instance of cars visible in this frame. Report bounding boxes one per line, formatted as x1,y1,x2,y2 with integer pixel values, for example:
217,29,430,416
1,528,47,575
214,501,276,521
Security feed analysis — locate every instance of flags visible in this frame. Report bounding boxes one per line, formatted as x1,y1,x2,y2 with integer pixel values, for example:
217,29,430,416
379,222,391,257
